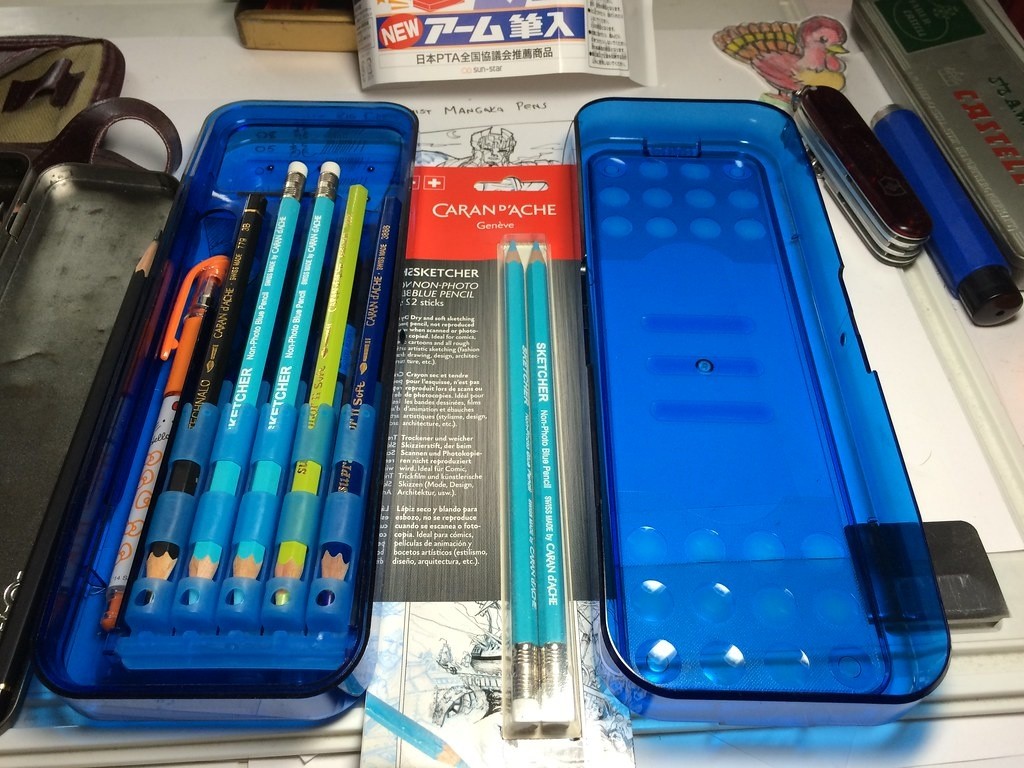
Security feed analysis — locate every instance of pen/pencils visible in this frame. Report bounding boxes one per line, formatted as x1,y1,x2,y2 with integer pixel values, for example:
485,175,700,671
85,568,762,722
0,227,162,697
50,161,404,632
508,237,574,734
365,692,469,767
